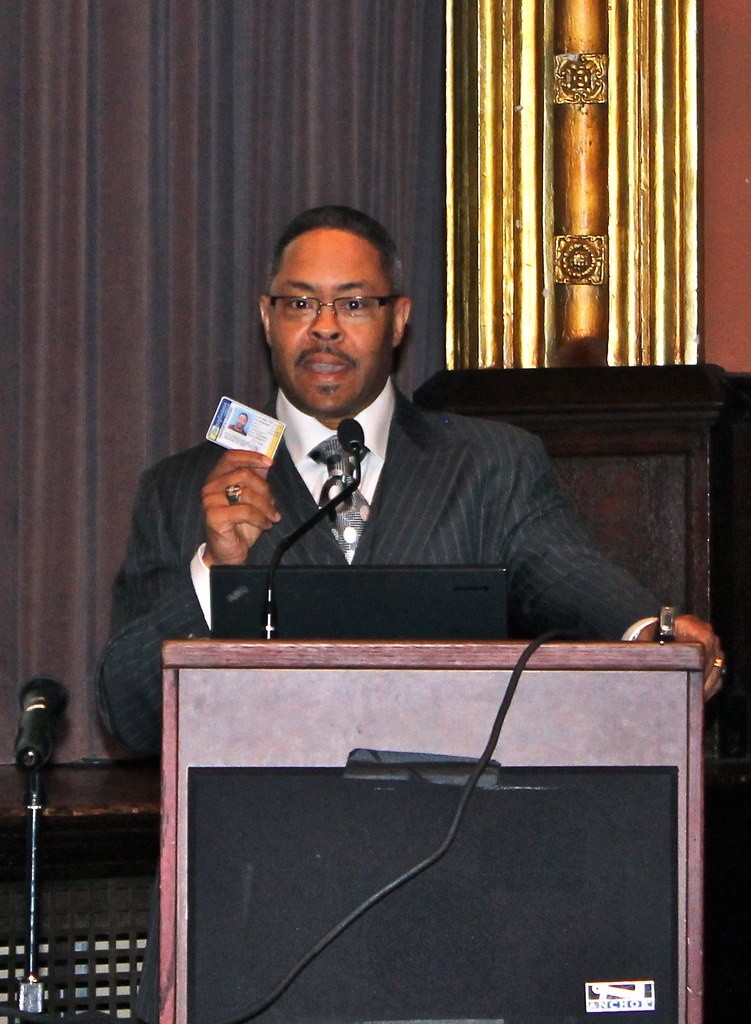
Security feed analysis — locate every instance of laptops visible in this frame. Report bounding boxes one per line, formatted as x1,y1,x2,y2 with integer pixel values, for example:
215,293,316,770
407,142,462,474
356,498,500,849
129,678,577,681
210,563,510,641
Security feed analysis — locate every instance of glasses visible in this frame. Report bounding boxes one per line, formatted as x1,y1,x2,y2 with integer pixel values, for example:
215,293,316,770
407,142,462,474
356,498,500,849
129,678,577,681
266,295,401,322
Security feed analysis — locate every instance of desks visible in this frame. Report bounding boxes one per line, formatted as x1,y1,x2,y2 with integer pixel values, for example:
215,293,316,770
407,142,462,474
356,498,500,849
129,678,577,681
0,753,164,831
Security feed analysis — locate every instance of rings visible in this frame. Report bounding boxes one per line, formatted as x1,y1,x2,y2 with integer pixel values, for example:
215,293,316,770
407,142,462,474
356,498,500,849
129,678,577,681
224,485,242,504
713,657,723,667
721,666,727,674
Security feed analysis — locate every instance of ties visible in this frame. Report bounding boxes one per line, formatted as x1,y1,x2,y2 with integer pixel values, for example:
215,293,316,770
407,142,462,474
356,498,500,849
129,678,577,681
307,433,372,566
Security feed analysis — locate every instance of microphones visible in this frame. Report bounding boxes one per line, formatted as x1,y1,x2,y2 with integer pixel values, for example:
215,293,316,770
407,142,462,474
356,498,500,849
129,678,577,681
260,417,365,641
13,675,70,771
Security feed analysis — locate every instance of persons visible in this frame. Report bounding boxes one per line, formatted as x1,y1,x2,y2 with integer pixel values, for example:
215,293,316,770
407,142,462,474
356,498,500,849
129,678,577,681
229,413,248,435
96,206,734,1024
